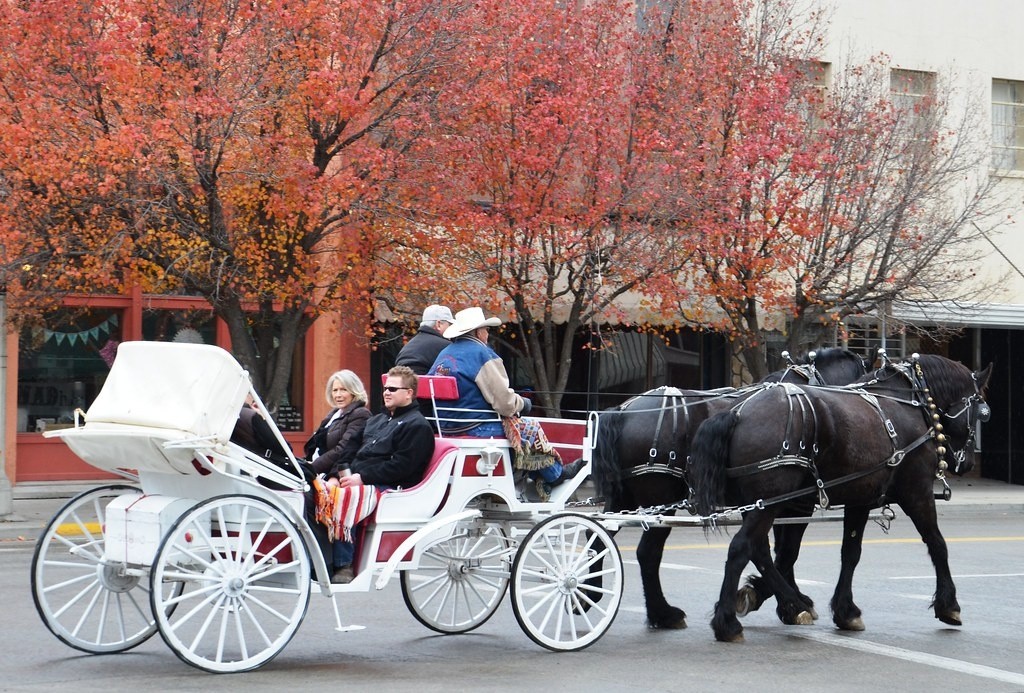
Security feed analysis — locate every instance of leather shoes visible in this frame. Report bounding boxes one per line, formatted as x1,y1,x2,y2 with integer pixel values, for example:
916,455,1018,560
546,458,583,488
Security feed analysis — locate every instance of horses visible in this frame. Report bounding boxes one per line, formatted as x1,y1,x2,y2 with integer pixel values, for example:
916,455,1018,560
567,346,879,629
691,353,993,643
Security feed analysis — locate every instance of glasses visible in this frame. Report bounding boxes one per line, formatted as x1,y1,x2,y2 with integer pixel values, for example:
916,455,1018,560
482,325,490,332
383,386,412,392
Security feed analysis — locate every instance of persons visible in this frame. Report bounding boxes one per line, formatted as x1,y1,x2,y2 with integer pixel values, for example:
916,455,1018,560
320,366,435,582
303,367,371,477
396,304,456,436
234,389,332,575
426,307,587,489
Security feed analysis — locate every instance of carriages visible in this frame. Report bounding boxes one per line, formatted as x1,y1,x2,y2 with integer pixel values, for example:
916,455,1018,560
30,341,993,675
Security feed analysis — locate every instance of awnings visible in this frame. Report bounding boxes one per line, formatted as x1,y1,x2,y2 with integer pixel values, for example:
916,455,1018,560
373,218,787,333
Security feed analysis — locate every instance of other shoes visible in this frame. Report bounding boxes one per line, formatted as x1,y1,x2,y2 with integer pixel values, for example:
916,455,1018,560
331,566,354,583
313,567,334,581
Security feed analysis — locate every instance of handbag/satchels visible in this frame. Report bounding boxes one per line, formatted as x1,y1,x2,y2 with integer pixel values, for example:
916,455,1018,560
287,461,317,483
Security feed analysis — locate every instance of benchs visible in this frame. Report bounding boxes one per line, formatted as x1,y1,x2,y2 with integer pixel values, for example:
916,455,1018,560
146,436,305,523
380,373,512,448
358,437,460,525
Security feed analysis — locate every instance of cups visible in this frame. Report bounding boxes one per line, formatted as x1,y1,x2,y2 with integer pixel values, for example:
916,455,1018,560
337,463,351,478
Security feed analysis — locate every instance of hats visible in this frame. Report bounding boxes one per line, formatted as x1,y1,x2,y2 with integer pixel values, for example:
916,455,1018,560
422,304,456,324
442,307,503,339
